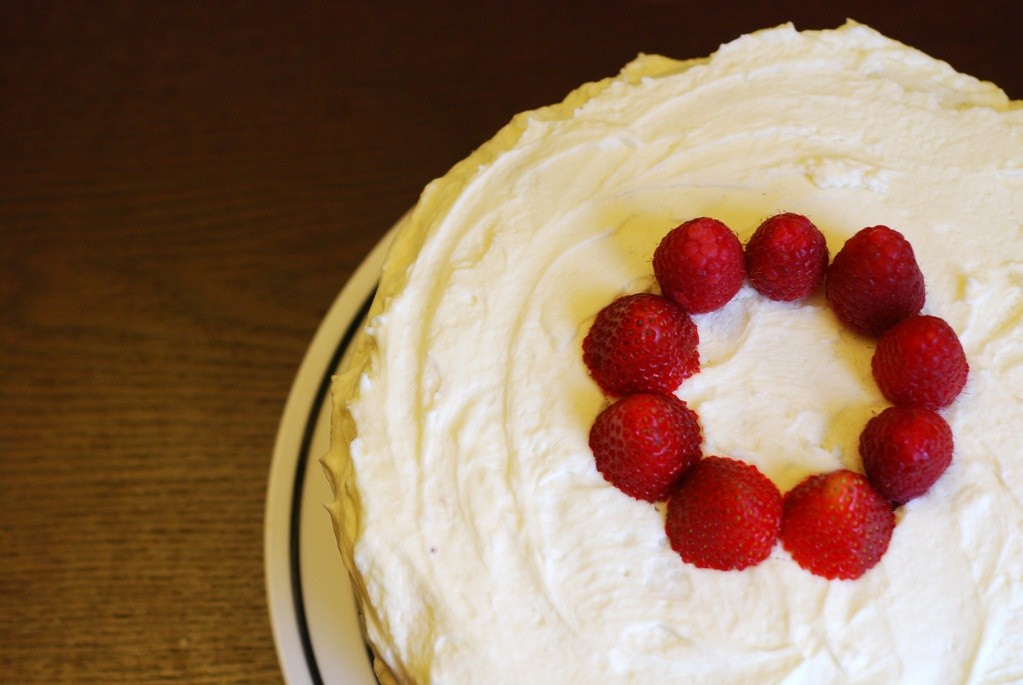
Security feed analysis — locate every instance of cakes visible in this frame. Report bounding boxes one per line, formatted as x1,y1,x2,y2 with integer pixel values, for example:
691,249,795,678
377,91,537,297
324,15,1023,685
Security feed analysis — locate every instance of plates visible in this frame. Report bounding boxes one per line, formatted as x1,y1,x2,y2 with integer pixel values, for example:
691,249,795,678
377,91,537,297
265,232,383,685
